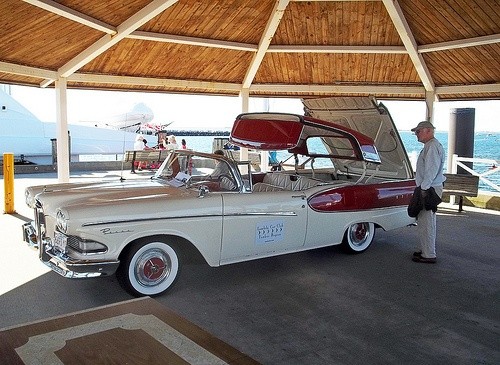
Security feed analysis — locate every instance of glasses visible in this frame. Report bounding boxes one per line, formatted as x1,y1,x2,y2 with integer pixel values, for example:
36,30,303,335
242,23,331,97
415,130,424,135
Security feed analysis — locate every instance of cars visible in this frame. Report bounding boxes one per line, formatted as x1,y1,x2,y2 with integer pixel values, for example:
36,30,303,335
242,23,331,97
21,94,419,300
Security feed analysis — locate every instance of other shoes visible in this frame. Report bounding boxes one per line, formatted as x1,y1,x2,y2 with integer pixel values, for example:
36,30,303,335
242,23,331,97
413,251,422,256
411,255,437,264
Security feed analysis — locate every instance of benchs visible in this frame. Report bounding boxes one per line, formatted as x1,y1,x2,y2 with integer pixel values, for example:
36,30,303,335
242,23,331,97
121,152,192,179
413,172,480,213
215,173,327,193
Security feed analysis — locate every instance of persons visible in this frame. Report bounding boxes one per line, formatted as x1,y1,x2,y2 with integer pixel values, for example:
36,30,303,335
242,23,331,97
222,138,240,149
210,149,244,190
133,133,192,174
269,150,281,170
407,121,446,264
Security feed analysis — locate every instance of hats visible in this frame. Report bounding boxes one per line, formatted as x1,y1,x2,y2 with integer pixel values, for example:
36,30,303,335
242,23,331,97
411,121,434,131
